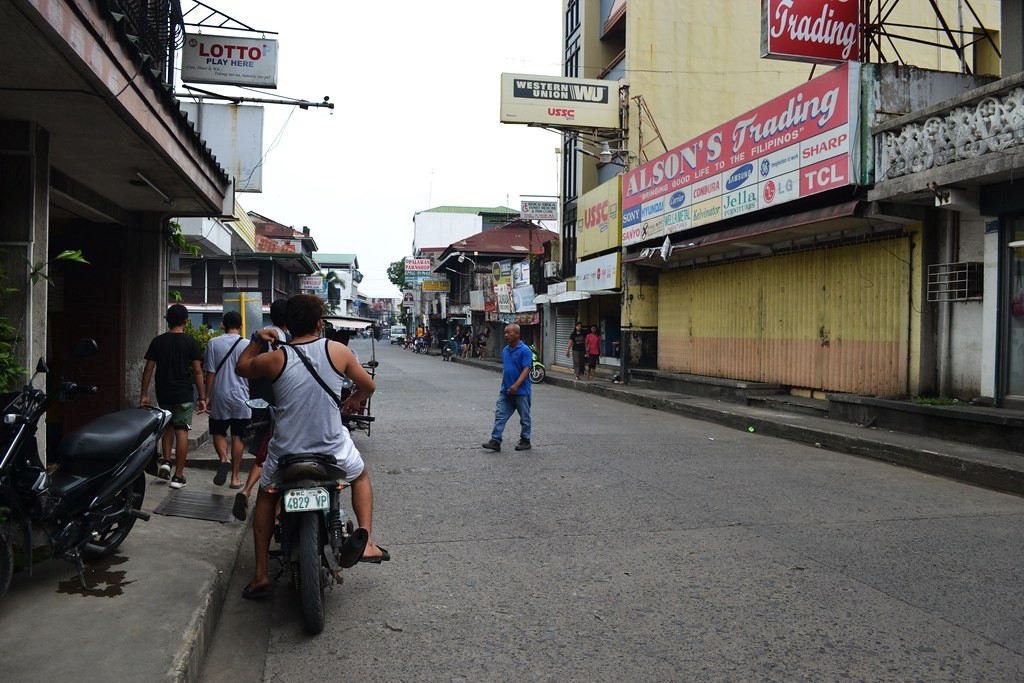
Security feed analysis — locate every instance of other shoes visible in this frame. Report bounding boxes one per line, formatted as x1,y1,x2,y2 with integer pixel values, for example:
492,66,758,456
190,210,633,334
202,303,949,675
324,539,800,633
576,376,580,381
482,437,502,452
516,439,532,451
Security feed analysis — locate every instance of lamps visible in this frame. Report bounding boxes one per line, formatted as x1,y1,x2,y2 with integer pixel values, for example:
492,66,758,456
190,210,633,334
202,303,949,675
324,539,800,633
446,267,472,276
458,252,477,271
640,235,699,262
136,169,176,208
599,141,639,170
573,146,628,172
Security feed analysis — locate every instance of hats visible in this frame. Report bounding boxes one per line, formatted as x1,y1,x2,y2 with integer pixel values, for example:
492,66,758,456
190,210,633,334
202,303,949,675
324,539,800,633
167,303,188,323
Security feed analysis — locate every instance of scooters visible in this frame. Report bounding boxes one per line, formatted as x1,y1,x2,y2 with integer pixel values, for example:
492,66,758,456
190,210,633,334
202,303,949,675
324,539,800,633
528,344,546,384
441,340,452,361
403,338,430,354
0,358,172,599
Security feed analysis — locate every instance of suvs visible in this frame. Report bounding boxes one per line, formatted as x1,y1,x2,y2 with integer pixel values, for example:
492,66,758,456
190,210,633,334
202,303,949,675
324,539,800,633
383,328,391,335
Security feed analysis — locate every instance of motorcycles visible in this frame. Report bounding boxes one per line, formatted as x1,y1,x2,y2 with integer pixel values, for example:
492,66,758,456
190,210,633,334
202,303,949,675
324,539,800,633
244,361,382,637
363,332,373,339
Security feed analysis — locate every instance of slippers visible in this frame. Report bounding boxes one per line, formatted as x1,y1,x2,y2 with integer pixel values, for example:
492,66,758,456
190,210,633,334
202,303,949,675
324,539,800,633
240,581,272,600
213,460,232,486
232,480,244,489
232,493,246,522
360,545,392,564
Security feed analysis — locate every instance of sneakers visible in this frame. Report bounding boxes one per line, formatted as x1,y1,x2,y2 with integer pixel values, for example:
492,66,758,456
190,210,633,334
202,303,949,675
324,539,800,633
169,474,187,489
159,461,172,481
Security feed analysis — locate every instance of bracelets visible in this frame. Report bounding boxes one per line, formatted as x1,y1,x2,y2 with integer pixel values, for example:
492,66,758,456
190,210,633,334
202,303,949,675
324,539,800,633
196,398,205,401
251,331,266,344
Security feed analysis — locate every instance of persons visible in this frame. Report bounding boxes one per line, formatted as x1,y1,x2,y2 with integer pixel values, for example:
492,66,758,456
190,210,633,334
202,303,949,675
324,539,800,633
234,294,391,597
231,298,292,522
332,330,359,363
566,322,602,380
481,323,533,452
140,304,207,487
207,324,214,334
417,325,431,340
202,311,252,489
454,325,472,359
478,327,490,361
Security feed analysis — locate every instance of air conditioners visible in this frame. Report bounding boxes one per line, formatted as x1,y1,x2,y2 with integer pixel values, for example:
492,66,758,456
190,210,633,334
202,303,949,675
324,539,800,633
544,262,557,277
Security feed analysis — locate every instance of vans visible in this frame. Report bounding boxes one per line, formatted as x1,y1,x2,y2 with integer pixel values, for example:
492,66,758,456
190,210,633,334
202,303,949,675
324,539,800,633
390,326,407,344
